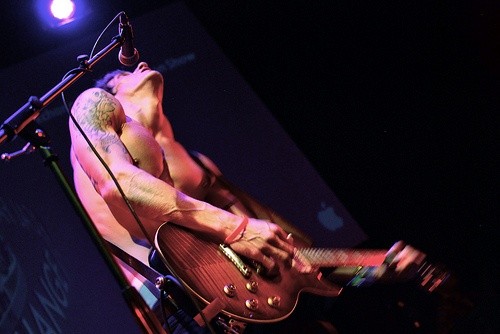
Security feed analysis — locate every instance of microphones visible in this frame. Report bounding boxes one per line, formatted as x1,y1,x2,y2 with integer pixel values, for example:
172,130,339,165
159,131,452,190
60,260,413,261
118,11,139,67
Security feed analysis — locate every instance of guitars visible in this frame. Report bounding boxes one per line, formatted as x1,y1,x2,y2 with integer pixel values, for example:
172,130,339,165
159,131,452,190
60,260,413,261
148,221,446,334
